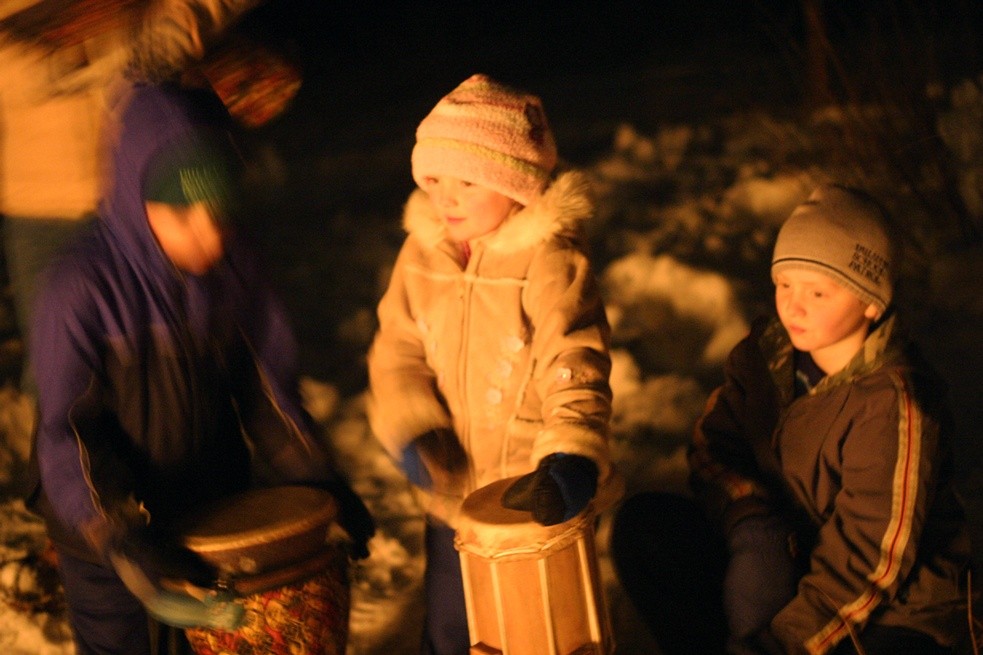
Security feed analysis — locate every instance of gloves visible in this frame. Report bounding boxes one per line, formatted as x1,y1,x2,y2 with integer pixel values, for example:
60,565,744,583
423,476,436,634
112,518,228,594
316,470,376,560
502,452,599,525
404,429,467,479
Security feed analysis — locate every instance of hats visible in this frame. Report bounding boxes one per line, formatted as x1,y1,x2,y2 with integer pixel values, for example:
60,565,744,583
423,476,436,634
411,75,558,207
768,184,897,318
141,148,240,206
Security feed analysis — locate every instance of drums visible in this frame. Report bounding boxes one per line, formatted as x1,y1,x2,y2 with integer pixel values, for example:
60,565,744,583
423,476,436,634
452,473,617,655
176,483,352,655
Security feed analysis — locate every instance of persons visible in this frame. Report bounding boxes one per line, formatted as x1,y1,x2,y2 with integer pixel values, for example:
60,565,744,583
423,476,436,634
367,73,635,655
24,78,379,654
686,182,983,655
1,1,314,407
